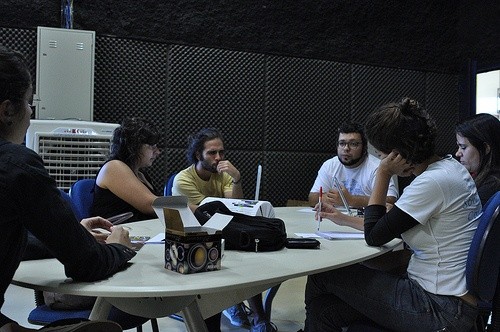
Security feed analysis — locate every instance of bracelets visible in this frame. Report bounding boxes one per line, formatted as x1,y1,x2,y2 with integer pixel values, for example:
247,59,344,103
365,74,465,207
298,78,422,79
232,177,242,184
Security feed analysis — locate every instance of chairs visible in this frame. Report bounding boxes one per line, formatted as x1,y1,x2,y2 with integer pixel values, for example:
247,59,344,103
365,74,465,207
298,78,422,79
452,190,500,332
28,178,160,332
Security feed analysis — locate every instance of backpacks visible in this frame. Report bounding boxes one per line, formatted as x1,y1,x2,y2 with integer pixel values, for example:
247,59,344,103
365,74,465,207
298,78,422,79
193,201,287,253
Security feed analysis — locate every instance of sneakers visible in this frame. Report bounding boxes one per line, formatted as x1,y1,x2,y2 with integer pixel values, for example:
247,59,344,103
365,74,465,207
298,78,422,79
223,302,253,329
248,315,279,332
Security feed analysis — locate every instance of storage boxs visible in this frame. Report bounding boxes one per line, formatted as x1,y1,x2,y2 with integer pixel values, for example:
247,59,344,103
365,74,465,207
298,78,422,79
150,195,234,276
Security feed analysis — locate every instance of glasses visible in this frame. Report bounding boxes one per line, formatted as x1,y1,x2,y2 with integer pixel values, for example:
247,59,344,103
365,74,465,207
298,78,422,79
7,93,41,106
336,140,363,149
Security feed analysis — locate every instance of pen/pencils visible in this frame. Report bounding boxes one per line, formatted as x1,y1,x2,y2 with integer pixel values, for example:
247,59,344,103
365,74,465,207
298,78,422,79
317,186,322,231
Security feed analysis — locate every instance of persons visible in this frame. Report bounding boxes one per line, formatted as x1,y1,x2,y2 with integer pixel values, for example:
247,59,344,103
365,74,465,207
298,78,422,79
84,117,223,332
301,97,485,332
170,127,281,332
0,45,137,332
308,117,402,273
451,112,500,242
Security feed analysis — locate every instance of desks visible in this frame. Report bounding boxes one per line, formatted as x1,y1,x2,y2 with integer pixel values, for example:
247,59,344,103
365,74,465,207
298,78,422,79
10,202,405,332
